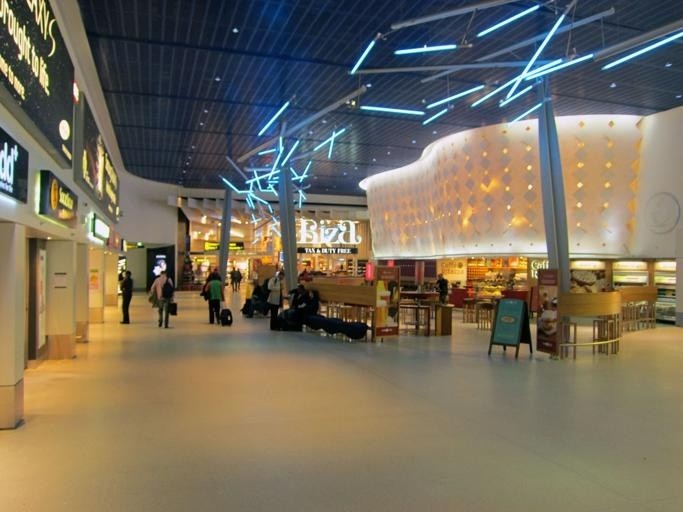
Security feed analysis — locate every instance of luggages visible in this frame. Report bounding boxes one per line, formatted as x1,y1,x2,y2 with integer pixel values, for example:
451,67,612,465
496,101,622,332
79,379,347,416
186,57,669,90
220,309,232,325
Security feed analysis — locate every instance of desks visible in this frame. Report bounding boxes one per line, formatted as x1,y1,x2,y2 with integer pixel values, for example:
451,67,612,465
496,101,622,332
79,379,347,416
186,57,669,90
401,290,439,335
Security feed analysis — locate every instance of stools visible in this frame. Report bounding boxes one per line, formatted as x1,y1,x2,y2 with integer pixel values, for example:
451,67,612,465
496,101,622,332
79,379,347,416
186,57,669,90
326,304,376,343
463,295,503,331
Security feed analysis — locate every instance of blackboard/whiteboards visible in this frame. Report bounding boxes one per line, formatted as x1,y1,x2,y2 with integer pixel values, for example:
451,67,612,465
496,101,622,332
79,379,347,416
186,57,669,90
491,298,524,346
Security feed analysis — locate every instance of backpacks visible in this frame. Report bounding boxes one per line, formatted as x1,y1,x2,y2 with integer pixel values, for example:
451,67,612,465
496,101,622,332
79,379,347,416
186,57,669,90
262,278,277,294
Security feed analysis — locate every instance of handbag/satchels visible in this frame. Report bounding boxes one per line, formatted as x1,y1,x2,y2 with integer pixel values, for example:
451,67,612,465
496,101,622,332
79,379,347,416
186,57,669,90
162,278,173,299
168,303,177,315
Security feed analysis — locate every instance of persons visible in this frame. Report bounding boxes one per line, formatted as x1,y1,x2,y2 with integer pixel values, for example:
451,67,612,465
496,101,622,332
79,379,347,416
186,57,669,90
119,271,133,323
267,270,320,331
236,268,242,291
436,273,448,302
229,267,238,292
204,275,226,326
151,272,174,329
206,268,221,281
